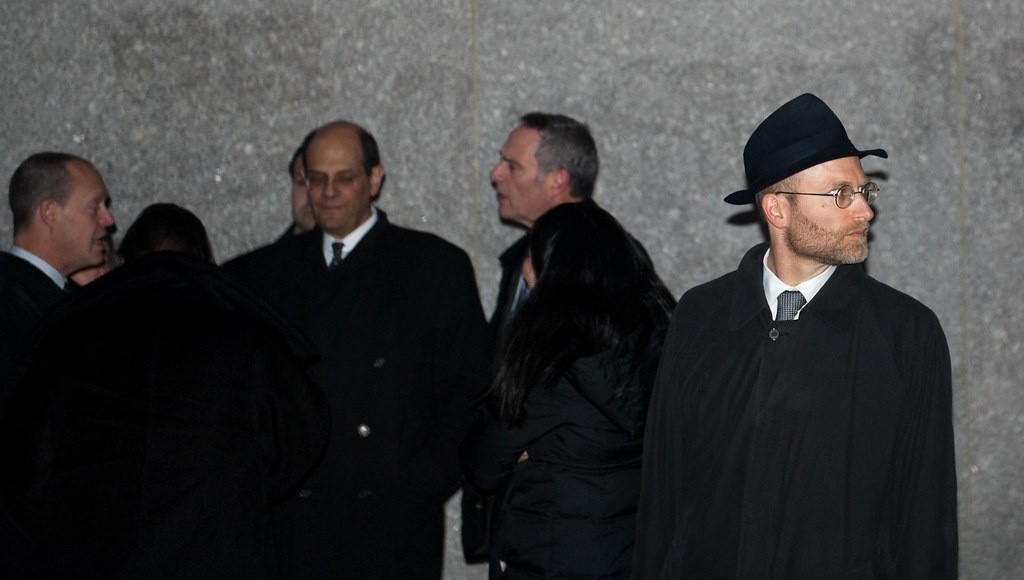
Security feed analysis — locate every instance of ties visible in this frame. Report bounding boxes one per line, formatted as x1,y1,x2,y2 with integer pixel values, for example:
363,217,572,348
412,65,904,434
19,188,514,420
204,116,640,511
332,242,345,267
775,289,805,322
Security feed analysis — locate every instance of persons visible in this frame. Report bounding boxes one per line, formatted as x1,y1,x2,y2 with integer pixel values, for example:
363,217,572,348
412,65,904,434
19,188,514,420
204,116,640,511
0,152,332,580
216,120,488,580
631,92,960,580
462,113,677,580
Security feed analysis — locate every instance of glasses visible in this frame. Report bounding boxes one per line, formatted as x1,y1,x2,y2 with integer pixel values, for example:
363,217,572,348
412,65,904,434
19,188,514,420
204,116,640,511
776,182,881,209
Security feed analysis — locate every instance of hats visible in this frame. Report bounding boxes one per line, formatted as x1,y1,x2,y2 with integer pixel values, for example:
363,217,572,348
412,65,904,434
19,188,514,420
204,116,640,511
723,93,886,205
